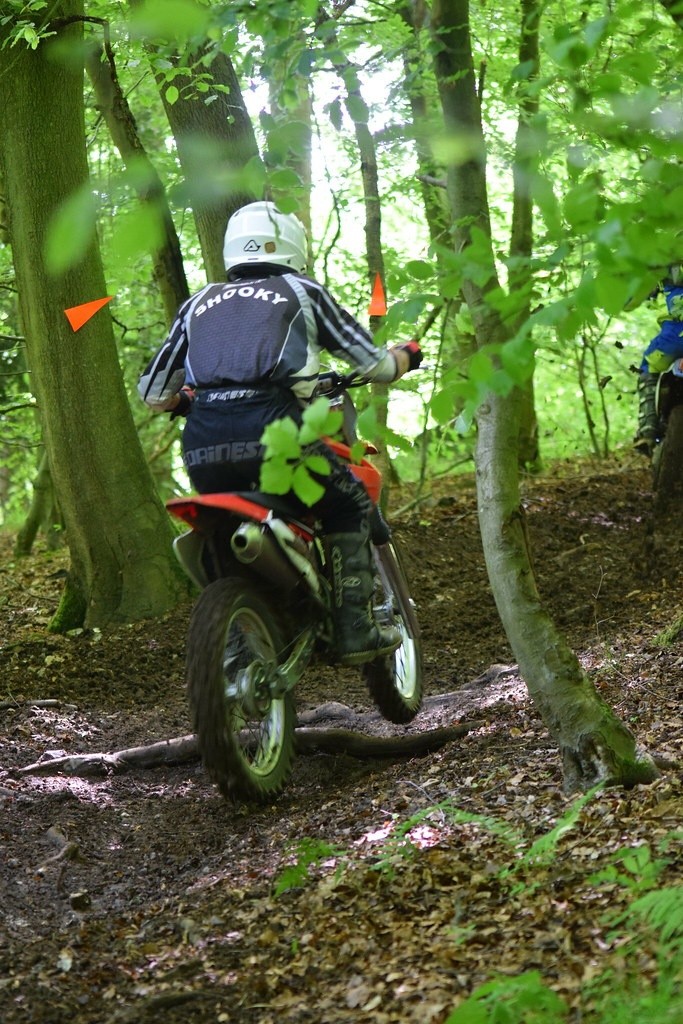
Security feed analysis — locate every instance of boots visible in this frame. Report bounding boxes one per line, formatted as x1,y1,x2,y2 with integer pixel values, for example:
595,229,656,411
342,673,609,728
322,532,403,666
633,372,660,457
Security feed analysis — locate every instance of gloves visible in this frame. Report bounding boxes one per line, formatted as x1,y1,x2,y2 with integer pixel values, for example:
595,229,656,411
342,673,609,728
395,341,423,372
169,383,198,421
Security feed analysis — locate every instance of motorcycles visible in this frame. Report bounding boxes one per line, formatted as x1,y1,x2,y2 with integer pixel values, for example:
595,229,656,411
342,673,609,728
164,351,430,814
641,359,683,510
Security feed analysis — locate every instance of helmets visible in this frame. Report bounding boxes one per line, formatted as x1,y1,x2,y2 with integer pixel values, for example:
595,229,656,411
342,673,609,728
223,201,308,282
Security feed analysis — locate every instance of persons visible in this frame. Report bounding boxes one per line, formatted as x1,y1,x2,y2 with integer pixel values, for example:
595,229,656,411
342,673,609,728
136,200,424,667
632,232,683,454
